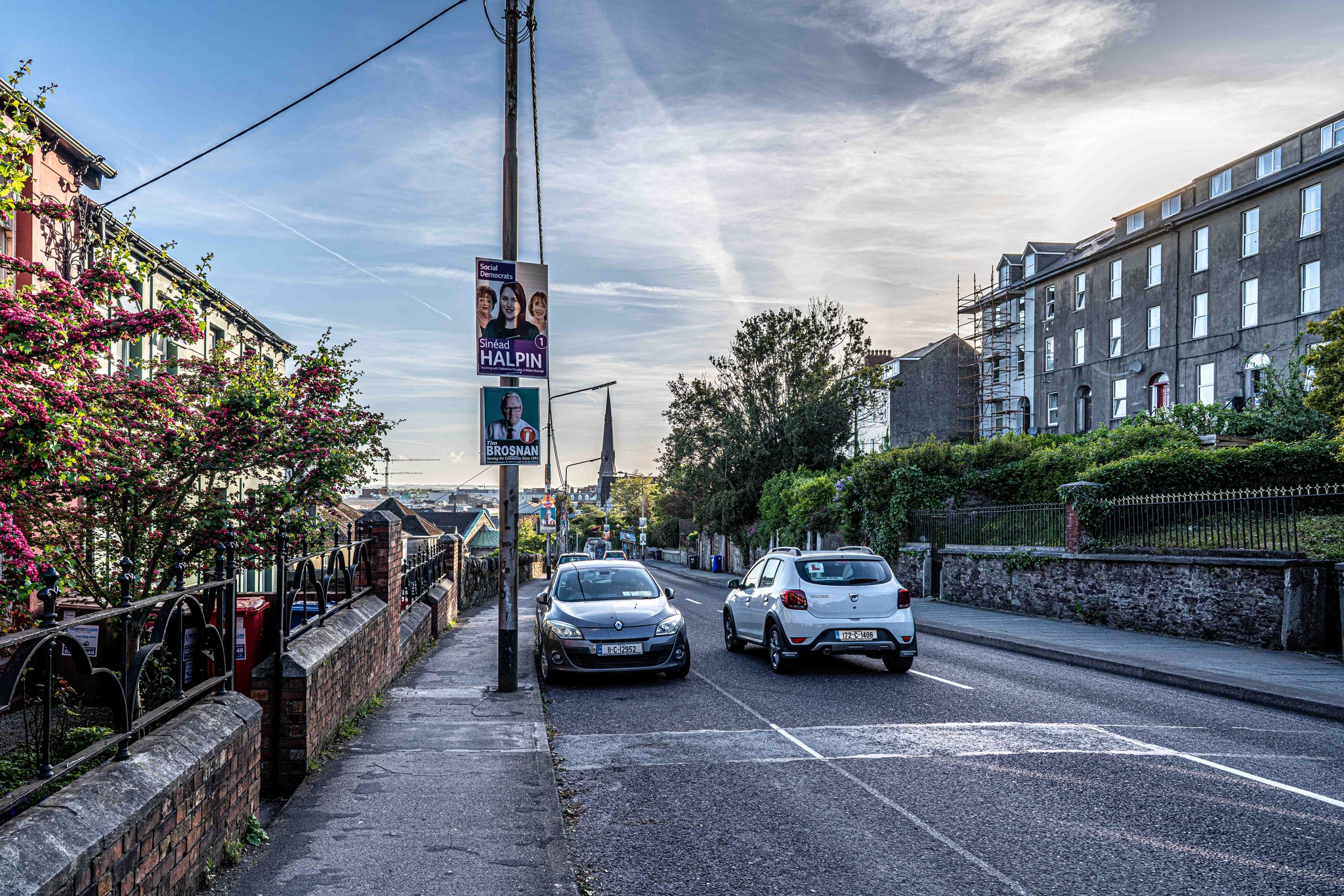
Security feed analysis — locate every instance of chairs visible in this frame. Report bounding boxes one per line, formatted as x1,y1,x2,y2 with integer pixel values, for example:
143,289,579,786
610,553,614,557
581,558,586,561
608,574,638,595
822,564,844,577
560,572,590,600
617,553,622,557
847,567,877,581
565,558,570,563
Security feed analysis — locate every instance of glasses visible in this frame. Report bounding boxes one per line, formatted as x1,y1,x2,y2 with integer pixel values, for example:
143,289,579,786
545,513,551,515
504,407,521,412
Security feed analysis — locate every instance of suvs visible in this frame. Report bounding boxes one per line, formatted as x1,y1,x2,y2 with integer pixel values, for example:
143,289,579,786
722,545,919,674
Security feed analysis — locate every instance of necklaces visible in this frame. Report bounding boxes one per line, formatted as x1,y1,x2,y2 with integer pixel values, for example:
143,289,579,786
478,314,492,324
532,318,545,330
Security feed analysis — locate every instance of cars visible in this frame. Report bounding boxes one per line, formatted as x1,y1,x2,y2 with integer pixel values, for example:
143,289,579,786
553,553,593,579
534,559,691,682
602,550,626,561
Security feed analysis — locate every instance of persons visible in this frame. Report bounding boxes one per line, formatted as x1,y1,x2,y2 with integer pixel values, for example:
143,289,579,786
484,391,539,440
606,538,609,541
540,509,556,525
545,467,550,485
604,527,609,533
640,520,646,527
640,536,646,543
476,281,547,343
562,522,569,527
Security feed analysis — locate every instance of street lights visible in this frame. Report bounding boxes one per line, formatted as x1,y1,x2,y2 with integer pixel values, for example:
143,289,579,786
541,377,616,578
562,456,606,553
618,471,645,562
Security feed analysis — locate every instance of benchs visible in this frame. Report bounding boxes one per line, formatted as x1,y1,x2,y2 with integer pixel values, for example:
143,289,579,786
581,579,614,589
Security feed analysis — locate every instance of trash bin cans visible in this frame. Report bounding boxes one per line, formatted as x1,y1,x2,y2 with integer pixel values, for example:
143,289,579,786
688,555,698,570
290,601,335,631
143,602,203,692
710,555,722,573
652,550,656,560
54,594,113,677
654,550,661,560
202,596,270,698
